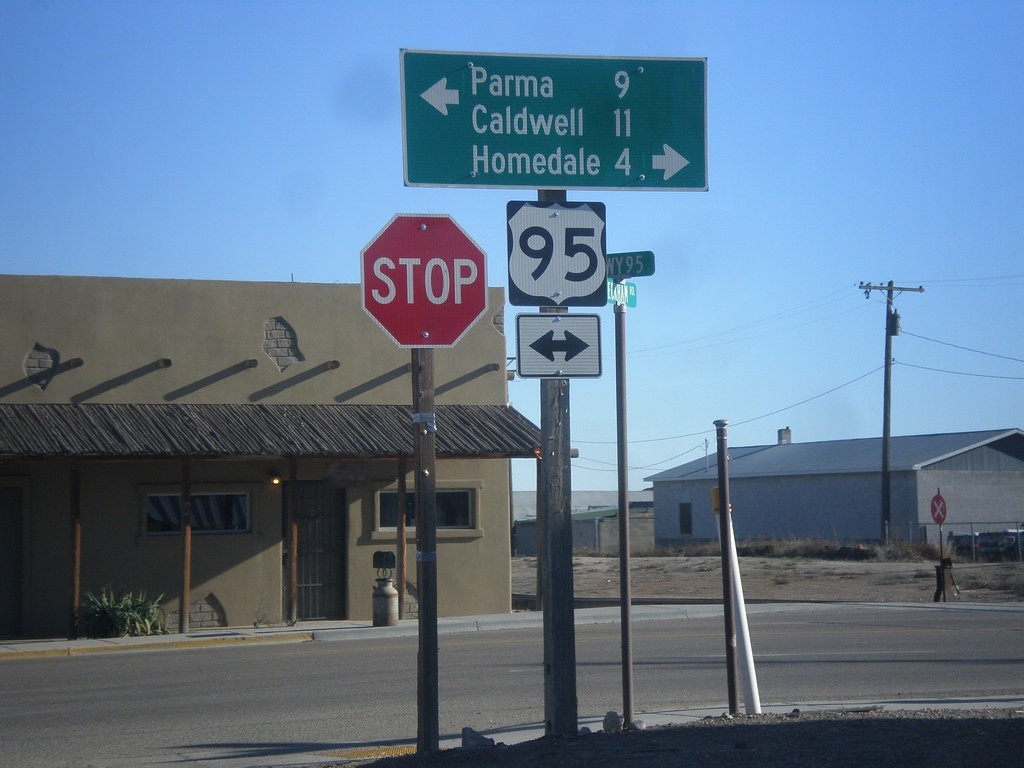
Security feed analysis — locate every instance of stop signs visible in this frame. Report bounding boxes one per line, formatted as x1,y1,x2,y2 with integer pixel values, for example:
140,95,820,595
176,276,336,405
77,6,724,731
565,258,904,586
360,212,489,349
